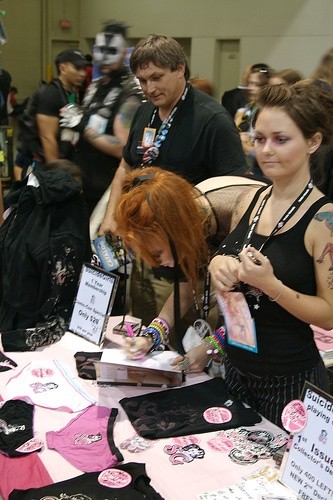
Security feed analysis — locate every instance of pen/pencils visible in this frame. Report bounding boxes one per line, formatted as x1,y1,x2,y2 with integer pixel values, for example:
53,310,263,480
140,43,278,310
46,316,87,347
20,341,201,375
126,322,144,361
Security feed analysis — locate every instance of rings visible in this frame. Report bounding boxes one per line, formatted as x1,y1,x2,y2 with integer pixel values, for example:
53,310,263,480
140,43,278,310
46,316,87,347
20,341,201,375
179,359,189,370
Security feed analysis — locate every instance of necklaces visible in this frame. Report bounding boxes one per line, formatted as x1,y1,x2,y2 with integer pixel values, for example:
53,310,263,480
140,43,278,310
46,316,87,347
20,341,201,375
193,186,220,239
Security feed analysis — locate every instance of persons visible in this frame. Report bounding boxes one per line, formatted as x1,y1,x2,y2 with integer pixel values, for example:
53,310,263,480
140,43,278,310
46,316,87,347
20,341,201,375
113,167,268,373
98,34,250,355
0,52,95,125
219,50,333,202
187,78,213,96
59,24,149,218
206,83,333,435
44,159,83,195
25,49,93,169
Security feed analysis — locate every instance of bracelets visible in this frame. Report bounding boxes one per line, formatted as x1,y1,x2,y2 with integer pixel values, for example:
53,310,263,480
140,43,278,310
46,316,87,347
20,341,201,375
208,325,227,356
223,254,238,260
269,280,283,301
142,318,169,354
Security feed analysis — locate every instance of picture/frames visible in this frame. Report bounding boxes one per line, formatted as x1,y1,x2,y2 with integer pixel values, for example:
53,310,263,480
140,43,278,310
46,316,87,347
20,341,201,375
66,263,119,347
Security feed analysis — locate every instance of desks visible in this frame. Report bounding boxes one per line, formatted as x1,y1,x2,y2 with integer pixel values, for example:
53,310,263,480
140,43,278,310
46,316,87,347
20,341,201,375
1,314,293,500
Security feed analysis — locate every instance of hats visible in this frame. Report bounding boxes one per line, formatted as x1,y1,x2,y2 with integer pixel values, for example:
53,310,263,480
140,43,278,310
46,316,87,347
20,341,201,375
54,49,88,68
